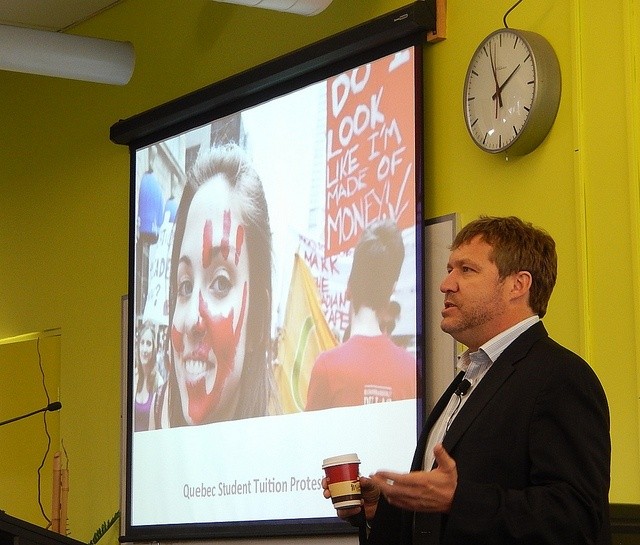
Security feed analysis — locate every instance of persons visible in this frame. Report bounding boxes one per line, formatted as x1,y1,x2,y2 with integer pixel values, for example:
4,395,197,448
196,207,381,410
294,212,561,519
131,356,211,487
305,218,416,412
166,144,272,427
135,322,164,431
322,216,611,545
134,145,165,324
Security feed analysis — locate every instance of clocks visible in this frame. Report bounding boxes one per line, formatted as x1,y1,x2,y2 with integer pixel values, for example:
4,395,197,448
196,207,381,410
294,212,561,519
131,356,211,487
462,28,561,160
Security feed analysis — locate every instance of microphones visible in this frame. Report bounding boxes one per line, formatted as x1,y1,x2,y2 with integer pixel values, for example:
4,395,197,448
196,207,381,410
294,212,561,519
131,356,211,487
445,377,471,437
0,402,62,426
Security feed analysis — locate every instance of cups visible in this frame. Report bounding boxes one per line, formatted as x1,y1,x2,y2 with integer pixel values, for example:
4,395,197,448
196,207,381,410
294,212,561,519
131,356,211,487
322,453,361,509
